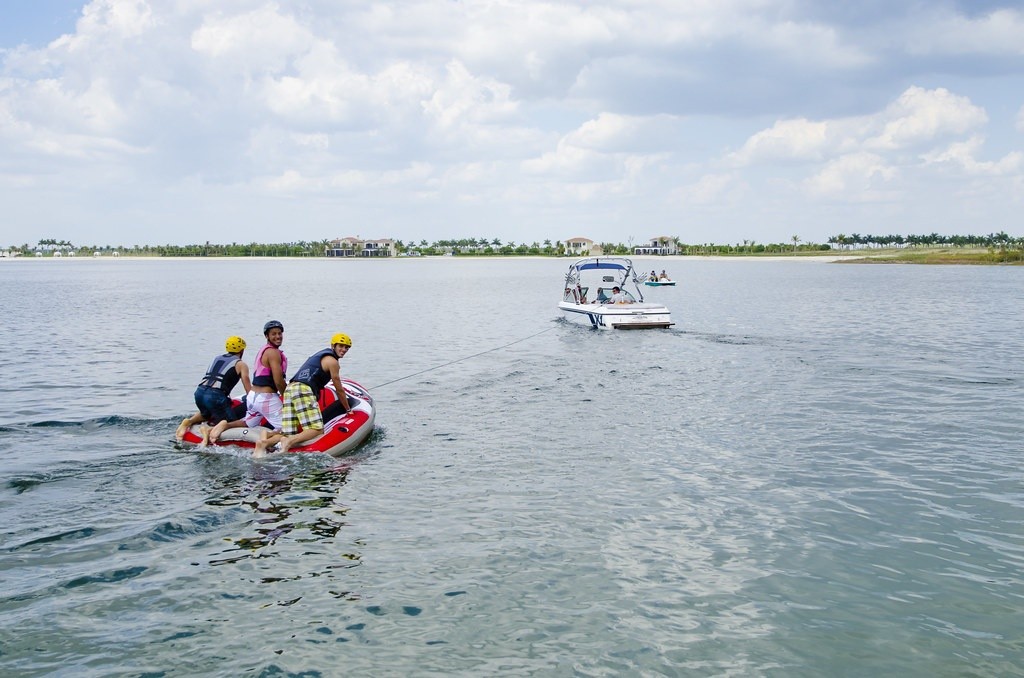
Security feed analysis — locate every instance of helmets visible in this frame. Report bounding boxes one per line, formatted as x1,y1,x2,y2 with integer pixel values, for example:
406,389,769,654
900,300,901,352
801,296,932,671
226,336,246,352
264,320,284,335
331,334,352,348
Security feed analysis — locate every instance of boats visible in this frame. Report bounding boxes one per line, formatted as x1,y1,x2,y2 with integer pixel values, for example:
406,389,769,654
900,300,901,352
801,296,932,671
558,257,676,330
644,278,675,286
179,376,375,457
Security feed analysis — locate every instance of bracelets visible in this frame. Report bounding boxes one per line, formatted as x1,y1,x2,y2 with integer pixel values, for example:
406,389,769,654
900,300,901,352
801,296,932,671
346,409,351,412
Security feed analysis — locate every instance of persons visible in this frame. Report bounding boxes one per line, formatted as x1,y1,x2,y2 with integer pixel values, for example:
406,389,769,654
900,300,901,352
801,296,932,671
650,270,655,281
254,334,354,457
608,286,625,304
176,336,251,446
660,270,670,281
591,288,608,303
209,320,288,450
